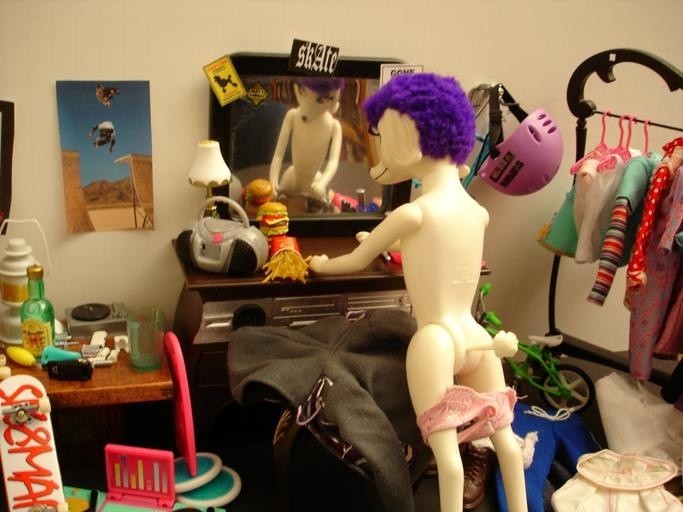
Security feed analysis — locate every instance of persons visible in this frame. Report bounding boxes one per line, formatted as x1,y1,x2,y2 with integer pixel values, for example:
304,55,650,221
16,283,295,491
96,84,121,107
87,120,116,153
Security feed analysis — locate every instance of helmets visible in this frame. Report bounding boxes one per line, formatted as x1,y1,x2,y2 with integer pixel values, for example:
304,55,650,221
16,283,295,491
474,80,566,197
